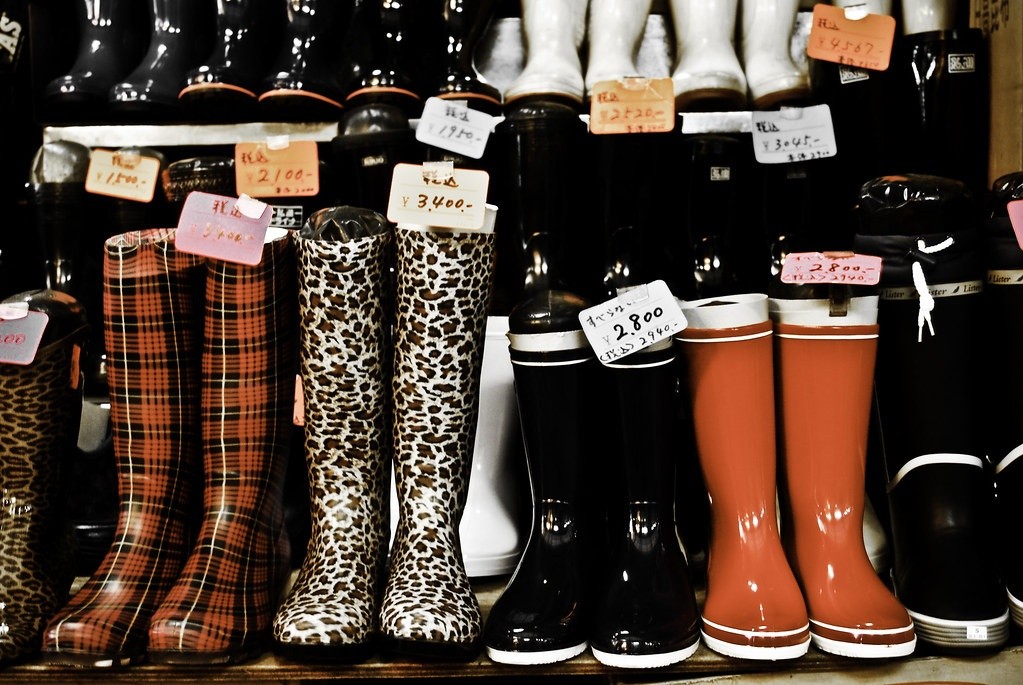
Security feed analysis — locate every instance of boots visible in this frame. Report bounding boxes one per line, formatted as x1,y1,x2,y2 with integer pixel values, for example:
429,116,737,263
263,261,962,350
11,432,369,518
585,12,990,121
0,0,1023,669
